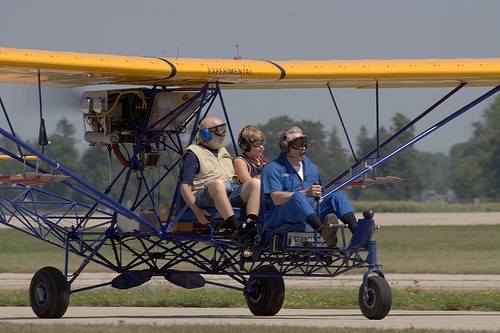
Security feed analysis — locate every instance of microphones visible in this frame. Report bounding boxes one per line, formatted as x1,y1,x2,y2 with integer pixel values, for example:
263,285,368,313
287,150,305,158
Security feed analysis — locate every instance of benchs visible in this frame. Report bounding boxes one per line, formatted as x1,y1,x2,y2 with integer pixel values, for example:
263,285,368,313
175,183,241,220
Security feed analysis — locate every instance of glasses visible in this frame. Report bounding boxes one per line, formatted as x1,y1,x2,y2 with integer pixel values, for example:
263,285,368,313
200,123,226,137
250,140,266,147
288,138,307,148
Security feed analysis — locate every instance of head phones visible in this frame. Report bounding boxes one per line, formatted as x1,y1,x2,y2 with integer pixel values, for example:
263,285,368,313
280,126,295,154
238,125,251,152
200,128,211,142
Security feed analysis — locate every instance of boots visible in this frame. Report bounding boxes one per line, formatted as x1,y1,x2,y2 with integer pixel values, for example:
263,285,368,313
321,214,338,246
225,214,259,242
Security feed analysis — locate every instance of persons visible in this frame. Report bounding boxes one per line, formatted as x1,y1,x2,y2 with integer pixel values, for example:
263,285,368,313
232,125,269,184
178,117,262,245
261,127,358,247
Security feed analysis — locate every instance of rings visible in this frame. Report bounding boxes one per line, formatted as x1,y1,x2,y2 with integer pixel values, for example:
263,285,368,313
317,192,320,195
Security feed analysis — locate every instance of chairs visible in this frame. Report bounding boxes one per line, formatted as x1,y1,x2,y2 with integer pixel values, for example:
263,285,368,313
258,202,314,232
